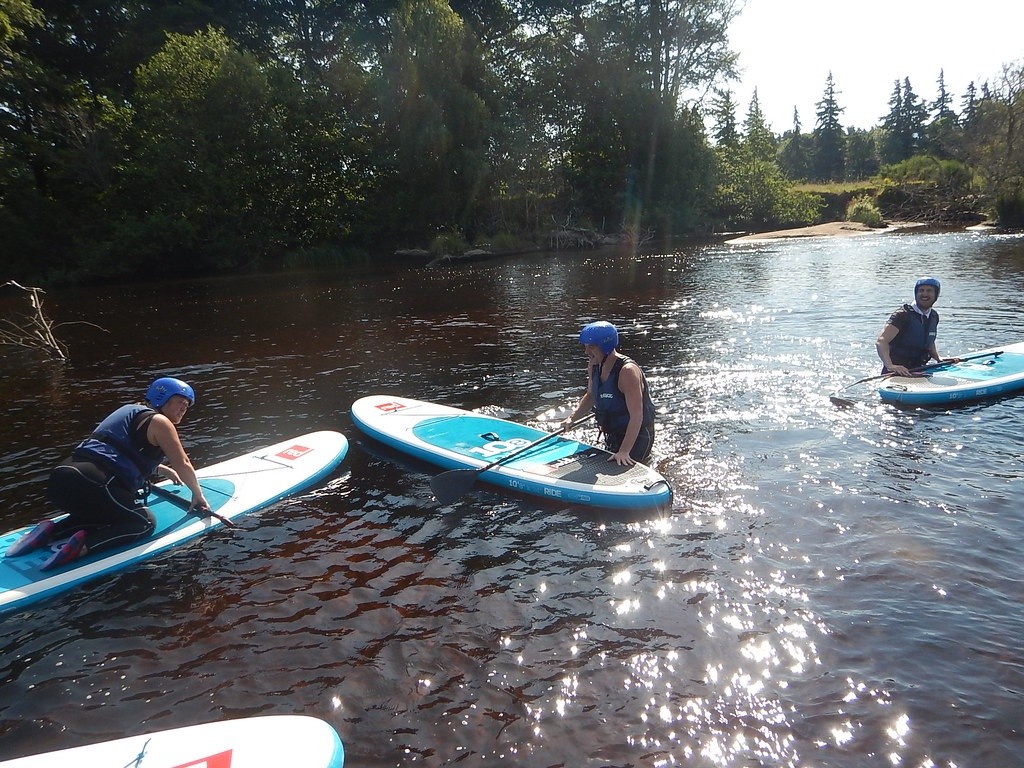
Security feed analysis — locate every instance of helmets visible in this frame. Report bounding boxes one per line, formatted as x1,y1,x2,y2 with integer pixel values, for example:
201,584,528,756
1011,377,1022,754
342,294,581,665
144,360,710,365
146,377,195,410
579,321,619,355
915,276,941,301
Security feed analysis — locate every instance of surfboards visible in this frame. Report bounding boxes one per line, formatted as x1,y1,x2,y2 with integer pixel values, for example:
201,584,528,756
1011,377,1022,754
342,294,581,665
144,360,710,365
877,340,1024,405
346,391,674,512
0,428,349,616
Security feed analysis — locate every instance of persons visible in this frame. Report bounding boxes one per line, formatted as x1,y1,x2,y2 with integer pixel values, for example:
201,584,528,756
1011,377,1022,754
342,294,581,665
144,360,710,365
876,279,961,377
555,320,655,466
6,378,211,572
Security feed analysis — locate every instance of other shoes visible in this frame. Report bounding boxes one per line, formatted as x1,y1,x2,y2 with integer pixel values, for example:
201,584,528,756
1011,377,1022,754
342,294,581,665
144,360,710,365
39,530,87,571
5,520,54,558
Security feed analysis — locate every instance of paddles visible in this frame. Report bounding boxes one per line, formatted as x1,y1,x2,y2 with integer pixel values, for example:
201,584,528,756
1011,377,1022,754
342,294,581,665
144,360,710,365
828,350,1008,391
136,473,235,532
426,409,600,507
